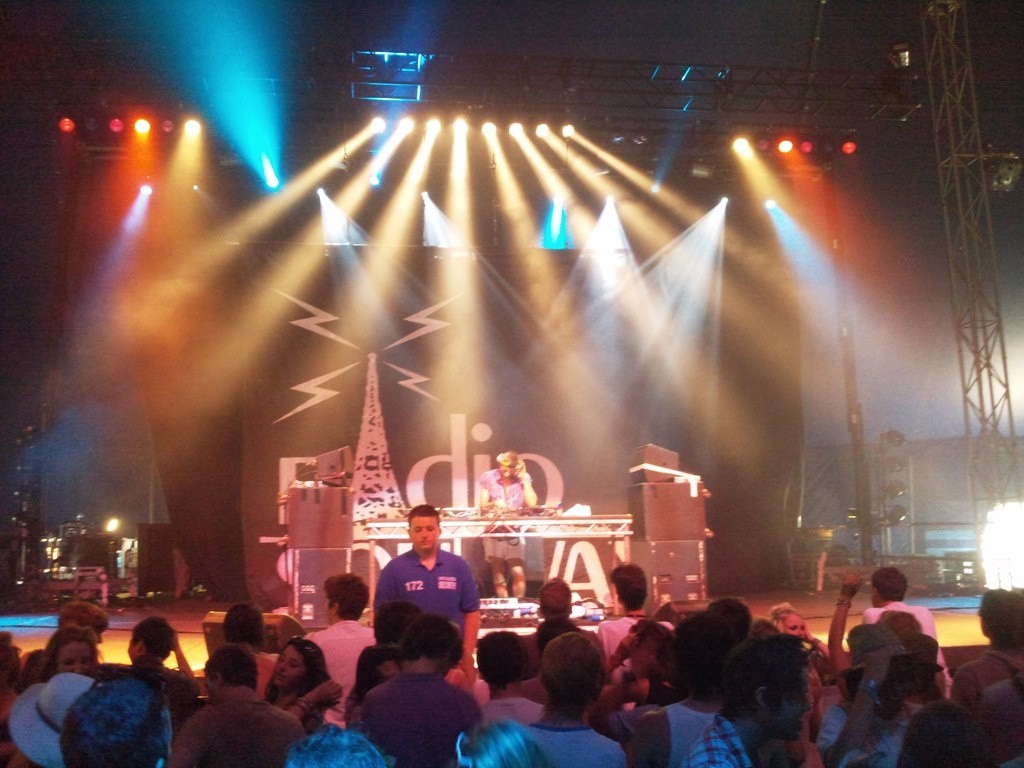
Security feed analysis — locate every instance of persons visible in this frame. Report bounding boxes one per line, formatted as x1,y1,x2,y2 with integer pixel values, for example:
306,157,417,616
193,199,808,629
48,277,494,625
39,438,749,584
479,451,537,599
374,505,479,654
1,566,1023,768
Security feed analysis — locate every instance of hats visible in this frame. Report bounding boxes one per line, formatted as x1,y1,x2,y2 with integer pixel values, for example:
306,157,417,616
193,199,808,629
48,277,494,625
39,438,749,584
879,610,938,664
8,672,103,768
834,621,923,676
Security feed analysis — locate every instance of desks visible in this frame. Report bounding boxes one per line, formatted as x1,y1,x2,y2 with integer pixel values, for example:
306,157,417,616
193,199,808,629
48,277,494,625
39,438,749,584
353,513,635,610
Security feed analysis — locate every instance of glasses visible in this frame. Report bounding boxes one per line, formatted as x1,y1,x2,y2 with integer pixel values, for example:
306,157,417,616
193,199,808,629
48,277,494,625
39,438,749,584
92,666,165,695
292,636,320,659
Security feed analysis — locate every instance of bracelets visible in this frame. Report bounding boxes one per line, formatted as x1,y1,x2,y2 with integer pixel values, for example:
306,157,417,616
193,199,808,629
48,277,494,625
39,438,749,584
812,638,821,646
836,598,851,607
295,697,312,713
860,679,877,697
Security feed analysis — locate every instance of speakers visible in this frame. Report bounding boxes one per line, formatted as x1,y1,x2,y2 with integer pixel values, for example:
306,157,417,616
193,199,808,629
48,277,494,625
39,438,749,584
201,611,308,653
625,444,708,618
286,446,354,629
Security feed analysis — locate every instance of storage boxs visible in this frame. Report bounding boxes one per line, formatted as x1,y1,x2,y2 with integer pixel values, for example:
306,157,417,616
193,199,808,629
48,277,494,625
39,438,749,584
287,485,357,550
291,550,352,626
626,480,716,541
630,541,710,612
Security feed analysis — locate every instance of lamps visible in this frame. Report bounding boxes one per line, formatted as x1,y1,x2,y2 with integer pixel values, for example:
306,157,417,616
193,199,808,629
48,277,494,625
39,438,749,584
161,112,175,133
755,132,772,151
886,456,905,473
632,443,681,482
633,126,653,146
608,125,631,148
58,108,81,132
654,597,712,626
840,135,858,156
109,111,127,132
85,108,105,132
297,446,356,486
201,610,308,659
882,479,910,499
800,136,819,156
886,503,908,525
878,429,907,448
